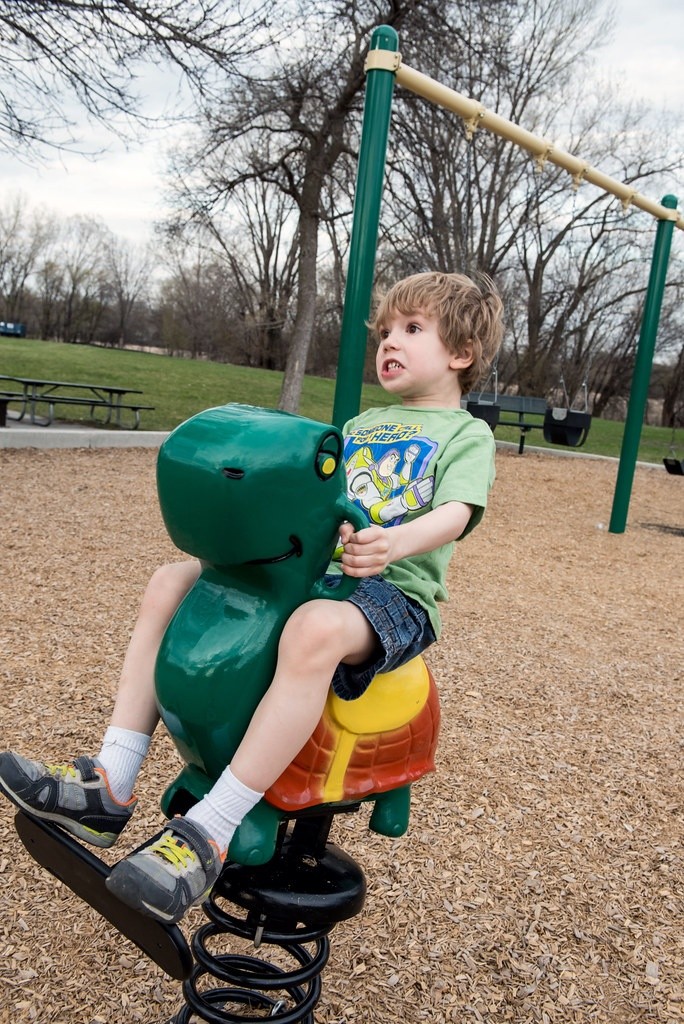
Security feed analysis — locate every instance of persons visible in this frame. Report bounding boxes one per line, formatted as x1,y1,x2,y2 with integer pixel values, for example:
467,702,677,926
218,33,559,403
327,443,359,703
1,272,504,921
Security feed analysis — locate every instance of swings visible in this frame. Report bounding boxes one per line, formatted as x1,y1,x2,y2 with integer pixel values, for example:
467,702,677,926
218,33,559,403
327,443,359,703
663,414,684,476
544,179,630,448
460,120,501,432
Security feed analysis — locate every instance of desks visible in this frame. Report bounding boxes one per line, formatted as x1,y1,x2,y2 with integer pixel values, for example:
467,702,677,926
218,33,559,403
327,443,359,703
1,377,144,429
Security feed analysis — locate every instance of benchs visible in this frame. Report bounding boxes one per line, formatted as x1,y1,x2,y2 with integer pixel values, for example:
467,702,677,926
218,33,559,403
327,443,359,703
465,390,549,454
0,389,154,428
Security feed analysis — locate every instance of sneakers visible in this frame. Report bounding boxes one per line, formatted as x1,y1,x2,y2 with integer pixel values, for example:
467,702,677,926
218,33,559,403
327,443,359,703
0,752,138,848
106,816,223,924
